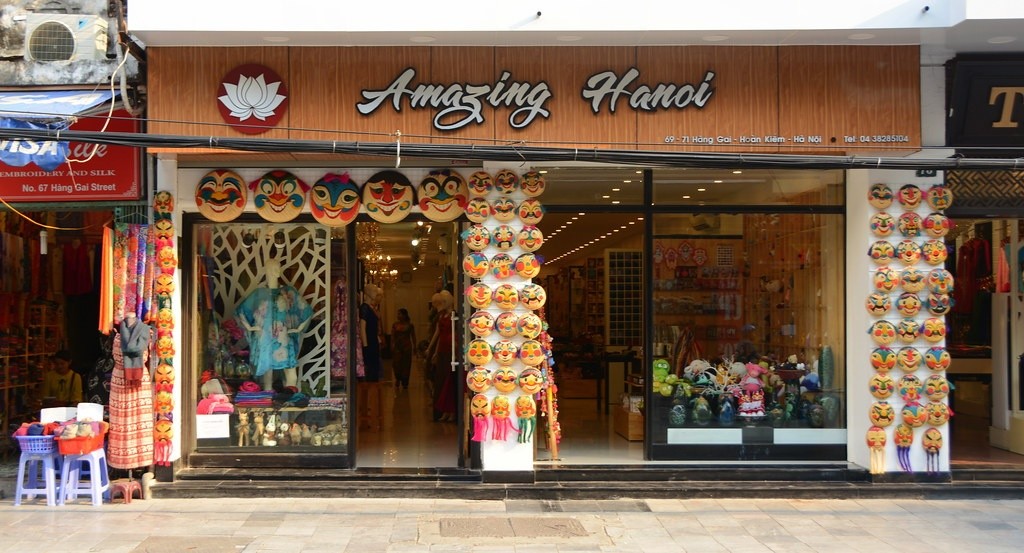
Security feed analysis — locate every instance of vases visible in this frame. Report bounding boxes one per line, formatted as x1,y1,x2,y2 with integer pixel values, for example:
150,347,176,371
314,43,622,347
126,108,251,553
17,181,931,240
237,357,248,375
214,348,224,375
225,356,236,376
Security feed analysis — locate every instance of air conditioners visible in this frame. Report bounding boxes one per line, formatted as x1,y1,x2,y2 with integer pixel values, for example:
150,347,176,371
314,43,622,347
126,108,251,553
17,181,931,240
24,13,108,60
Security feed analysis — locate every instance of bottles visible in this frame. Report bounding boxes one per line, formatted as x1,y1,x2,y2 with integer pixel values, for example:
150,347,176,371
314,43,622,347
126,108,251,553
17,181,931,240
666,379,687,425
819,390,840,427
716,392,734,427
817,346,834,388
691,394,715,427
235,360,251,380
807,399,826,427
799,396,811,419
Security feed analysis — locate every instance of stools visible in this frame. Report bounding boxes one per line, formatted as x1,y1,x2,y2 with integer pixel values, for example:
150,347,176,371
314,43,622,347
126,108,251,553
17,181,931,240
109,481,141,503
16,450,64,506
59,449,109,505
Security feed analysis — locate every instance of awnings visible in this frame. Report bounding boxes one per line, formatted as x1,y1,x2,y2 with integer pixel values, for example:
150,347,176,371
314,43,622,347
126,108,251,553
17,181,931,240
0,90,134,173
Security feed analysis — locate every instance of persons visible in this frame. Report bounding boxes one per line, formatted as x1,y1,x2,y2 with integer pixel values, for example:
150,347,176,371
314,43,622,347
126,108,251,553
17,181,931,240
36,350,83,408
424,290,457,423
735,324,768,364
106,312,153,468
360,283,384,432
391,308,417,400
234,258,314,391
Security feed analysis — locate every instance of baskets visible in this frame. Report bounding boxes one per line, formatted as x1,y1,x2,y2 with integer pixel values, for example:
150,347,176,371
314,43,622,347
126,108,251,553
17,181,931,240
16,434,57,455
53,431,106,455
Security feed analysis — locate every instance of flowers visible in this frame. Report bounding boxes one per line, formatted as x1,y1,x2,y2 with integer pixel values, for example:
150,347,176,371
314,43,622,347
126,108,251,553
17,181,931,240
206,320,251,356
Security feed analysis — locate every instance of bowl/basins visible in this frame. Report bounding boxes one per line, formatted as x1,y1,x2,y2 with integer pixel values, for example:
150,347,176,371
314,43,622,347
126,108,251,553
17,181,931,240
736,413,767,422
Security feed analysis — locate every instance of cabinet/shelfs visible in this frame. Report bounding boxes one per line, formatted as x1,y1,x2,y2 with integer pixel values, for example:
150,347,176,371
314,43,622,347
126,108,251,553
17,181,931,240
654,275,740,353
615,356,683,440
603,247,642,353
0,302,62,463
586,258,605,337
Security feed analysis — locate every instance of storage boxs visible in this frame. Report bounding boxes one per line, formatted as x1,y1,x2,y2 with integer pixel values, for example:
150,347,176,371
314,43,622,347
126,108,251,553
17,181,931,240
619,373,644,413
55,433,103,454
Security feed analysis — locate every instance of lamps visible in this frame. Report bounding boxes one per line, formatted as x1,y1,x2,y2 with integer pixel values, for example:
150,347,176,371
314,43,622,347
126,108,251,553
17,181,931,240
412,238,422,246
